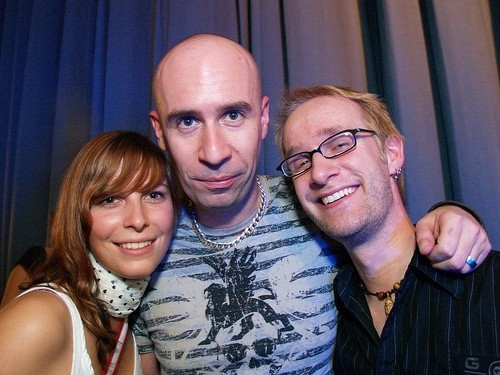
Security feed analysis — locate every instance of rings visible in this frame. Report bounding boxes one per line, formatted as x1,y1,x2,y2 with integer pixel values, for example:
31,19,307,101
466,255,477,269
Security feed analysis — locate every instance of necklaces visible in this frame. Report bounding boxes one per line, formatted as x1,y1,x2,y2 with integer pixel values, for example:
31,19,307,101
360,280,402,316
189,178,267,249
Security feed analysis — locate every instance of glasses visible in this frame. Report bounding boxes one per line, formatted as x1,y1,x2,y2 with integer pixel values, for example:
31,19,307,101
276,127,377,178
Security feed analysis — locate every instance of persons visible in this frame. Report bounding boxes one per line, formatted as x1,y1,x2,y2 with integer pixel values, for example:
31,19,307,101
0,33,492,375
0,130,187,375
272,86,500,375
